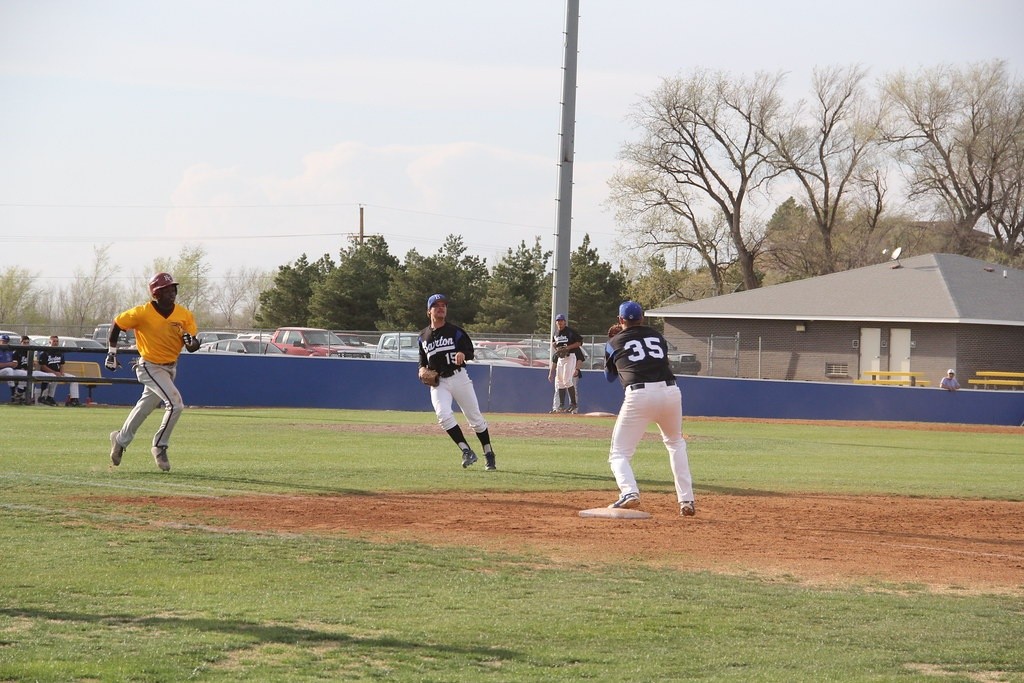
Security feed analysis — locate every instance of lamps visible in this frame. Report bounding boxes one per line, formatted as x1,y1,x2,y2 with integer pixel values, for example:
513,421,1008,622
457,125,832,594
796,324,805,332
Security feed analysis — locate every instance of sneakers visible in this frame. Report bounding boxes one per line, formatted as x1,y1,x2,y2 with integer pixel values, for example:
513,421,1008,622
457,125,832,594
567,405,578,412
550,406,567,414
152,445,171,471
462,451,478,468
483,452,496,470
109,431,125,466
608,492,640,509
680,501,695,517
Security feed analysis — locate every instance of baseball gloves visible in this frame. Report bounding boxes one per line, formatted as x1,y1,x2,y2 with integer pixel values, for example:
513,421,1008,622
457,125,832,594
608,324,623,338
419,369,440,387
555,346,569,359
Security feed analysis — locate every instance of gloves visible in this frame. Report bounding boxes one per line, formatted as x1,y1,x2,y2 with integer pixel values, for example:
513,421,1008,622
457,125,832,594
105,352,117,372
183,333,196,347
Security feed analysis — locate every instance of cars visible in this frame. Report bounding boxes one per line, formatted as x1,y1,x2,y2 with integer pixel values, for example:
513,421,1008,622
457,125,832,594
0,323,137,349
666,341,702,376
179,326,609,371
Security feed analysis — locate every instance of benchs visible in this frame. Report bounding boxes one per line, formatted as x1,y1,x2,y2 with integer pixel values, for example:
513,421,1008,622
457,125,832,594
853,371,932,387
11,360,113,405
968,371,1024,390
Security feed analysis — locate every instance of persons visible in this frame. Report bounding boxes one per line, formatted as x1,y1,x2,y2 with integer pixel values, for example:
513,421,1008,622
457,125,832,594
105,273,201,472
0,335,83,407
604,300,696,517
940,368,960,390
548,314,587,416
418,293,497,472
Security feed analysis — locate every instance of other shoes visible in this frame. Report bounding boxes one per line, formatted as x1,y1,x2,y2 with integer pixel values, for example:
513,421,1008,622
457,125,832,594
11,393,85,407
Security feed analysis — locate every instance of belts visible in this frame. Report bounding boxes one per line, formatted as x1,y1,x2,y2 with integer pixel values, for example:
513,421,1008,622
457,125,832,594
151,362,176,366
631,380,676,391
441,368,461,378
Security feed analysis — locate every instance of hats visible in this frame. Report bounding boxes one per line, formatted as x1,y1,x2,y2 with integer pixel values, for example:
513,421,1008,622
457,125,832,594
428,294,447,309
149,274,179,296
556,314,565,321
617,301,644,320
0,334,11,341
